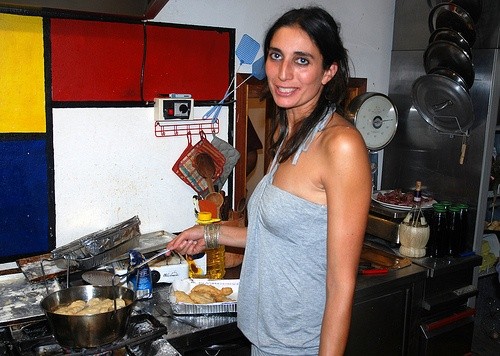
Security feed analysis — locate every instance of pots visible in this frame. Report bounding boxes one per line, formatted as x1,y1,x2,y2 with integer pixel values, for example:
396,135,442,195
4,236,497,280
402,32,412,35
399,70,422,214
39,285,138,350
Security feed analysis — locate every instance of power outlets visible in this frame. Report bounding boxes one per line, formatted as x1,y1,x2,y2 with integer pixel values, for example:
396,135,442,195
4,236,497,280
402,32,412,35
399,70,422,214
152,98,194,121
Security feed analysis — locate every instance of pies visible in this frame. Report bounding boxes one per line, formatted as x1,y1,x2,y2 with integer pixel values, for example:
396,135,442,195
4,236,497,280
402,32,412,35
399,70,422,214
54,296,125,315
174,283,237,305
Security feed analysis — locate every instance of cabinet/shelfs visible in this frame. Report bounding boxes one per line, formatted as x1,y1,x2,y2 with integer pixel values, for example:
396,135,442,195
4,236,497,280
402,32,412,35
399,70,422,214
380,0,500,356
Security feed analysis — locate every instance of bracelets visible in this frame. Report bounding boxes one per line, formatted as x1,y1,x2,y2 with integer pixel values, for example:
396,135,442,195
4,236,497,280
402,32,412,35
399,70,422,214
203,224,218,250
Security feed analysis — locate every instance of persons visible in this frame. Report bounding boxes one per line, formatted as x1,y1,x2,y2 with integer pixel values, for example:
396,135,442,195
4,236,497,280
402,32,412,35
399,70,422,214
166,6,371,356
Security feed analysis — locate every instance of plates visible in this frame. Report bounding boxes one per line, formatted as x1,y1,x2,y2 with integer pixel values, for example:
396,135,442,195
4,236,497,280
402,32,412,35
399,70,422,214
371,190,438,211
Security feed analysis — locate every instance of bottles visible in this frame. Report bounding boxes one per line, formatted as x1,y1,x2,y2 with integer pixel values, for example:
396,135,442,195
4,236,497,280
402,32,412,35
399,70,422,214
188,211,226,280
426,200,473,259
397,181,429,258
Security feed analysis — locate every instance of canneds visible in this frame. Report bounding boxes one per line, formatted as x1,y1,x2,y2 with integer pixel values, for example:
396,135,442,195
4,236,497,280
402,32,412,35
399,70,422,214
430,201,469,236
